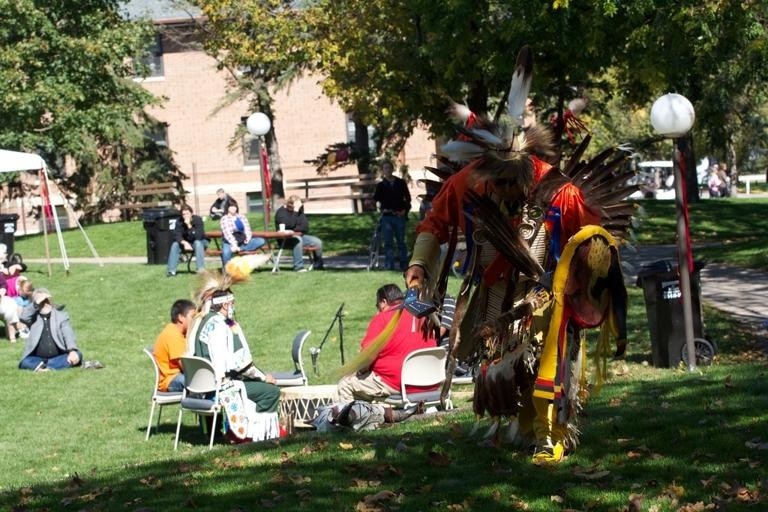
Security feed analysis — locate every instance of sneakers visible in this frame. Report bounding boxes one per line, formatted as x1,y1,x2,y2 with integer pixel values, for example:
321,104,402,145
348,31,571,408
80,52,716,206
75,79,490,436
531,439,564,466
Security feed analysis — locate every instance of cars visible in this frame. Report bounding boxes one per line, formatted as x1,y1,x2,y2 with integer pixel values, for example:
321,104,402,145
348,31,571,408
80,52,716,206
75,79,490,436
621,160,676,203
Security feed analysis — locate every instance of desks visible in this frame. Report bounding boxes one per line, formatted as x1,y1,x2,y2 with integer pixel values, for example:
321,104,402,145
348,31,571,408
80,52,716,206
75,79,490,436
203,230,294,272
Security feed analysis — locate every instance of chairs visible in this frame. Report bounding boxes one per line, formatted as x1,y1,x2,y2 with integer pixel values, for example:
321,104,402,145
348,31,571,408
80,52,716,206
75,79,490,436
446,377,475,411
174,356,222,450
271,330,312,386
375,347,453,412
143,348,209,441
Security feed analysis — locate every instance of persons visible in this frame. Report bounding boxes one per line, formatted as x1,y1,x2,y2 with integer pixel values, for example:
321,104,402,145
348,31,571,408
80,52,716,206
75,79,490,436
338,283,442,405
708,163,732,197
438,291,476,380
185,253,287,445
210,189,238,220
364,161,411,272
166,204,209,277
275,195,323,272
153,300,222,440
0,244,83,371
219,199,265,275
405,44,628,469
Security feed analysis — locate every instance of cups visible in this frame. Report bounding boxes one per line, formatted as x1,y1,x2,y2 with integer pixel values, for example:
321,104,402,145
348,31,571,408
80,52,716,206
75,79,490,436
279,223,285,232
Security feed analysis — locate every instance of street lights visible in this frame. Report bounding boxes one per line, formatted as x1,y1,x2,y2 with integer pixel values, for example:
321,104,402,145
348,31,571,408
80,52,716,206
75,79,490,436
246,111,272,233
649,90,697,373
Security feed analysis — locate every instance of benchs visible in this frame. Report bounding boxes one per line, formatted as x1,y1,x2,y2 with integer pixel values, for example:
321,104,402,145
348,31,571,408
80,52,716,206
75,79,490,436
113,181,177,222
259,245,320,271
286,174,380,213
180,250,259,273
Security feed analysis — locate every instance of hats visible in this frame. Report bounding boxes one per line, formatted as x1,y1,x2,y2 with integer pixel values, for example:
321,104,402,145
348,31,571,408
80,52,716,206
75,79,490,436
32,288,51,304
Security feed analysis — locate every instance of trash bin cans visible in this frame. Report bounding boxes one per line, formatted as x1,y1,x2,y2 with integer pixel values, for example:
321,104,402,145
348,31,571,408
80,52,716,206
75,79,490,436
636,259,719,369
0,214,19,257
143,208,180,264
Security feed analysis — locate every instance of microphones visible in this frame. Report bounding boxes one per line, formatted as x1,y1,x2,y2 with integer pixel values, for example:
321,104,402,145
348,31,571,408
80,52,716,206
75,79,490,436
309,347,317,374
